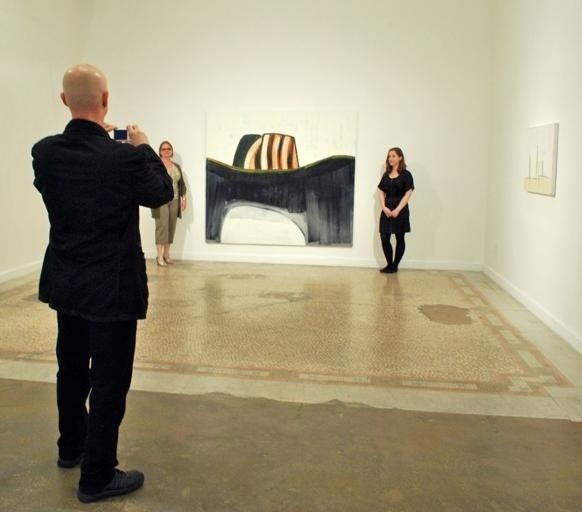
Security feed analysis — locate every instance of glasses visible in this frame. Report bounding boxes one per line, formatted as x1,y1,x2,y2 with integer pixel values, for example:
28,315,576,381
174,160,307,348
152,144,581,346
161,148,171,151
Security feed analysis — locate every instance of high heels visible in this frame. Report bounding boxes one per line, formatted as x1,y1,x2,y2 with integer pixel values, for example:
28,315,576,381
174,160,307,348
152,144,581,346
156,254,174,267
380,262,398,273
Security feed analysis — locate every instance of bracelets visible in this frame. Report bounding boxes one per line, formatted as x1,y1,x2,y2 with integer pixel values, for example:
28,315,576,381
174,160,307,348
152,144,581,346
182,197,186,201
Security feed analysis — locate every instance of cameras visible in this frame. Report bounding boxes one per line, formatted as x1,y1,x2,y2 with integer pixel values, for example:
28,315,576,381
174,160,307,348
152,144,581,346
112,128,129,142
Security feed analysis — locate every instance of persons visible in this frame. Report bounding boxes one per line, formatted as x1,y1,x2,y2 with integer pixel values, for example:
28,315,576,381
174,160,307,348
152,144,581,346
150,140,189,265
31,64,174,504
374,147,416,274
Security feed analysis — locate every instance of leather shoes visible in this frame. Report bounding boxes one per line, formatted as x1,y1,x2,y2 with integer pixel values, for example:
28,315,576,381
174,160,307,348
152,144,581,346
57,454,81,468
77,469,145,503
